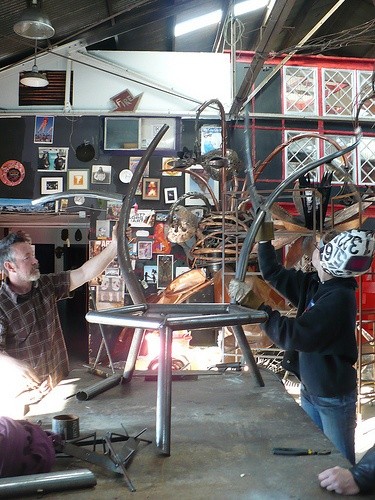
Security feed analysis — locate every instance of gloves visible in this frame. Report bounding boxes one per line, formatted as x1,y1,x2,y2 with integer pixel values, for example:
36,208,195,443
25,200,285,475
228,278,265,311
255,202,275,242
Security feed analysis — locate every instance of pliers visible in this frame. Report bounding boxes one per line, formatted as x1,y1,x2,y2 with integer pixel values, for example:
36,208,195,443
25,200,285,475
272,447,331,455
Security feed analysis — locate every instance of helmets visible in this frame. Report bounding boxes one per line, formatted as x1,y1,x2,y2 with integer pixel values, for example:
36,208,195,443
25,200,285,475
320,229,375,278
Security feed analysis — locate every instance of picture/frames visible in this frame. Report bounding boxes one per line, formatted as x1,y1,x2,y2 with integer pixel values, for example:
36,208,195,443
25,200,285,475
41,177,63,194
156,255,174,289
91,165,112,185
164,187,178,204
37,147,69,172
142,177,160,200
34,116,54,144
138,240,152,260
184,162,219,207
67,169,90,192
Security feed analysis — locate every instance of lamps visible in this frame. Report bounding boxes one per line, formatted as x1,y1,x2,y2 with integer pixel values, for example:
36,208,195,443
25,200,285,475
13,0,55,40
20,39,49,88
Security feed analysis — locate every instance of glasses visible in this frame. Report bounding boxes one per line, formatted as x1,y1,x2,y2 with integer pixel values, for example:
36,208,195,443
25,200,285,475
314,240,321,251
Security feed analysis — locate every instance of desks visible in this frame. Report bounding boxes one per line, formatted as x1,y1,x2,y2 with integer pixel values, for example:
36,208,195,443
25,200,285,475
20,368,359,500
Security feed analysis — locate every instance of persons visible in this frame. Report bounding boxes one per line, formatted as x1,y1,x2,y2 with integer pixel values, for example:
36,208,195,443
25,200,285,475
228,205,375,466
39,153,66,170
318,443,375,495
0,221,132,419
